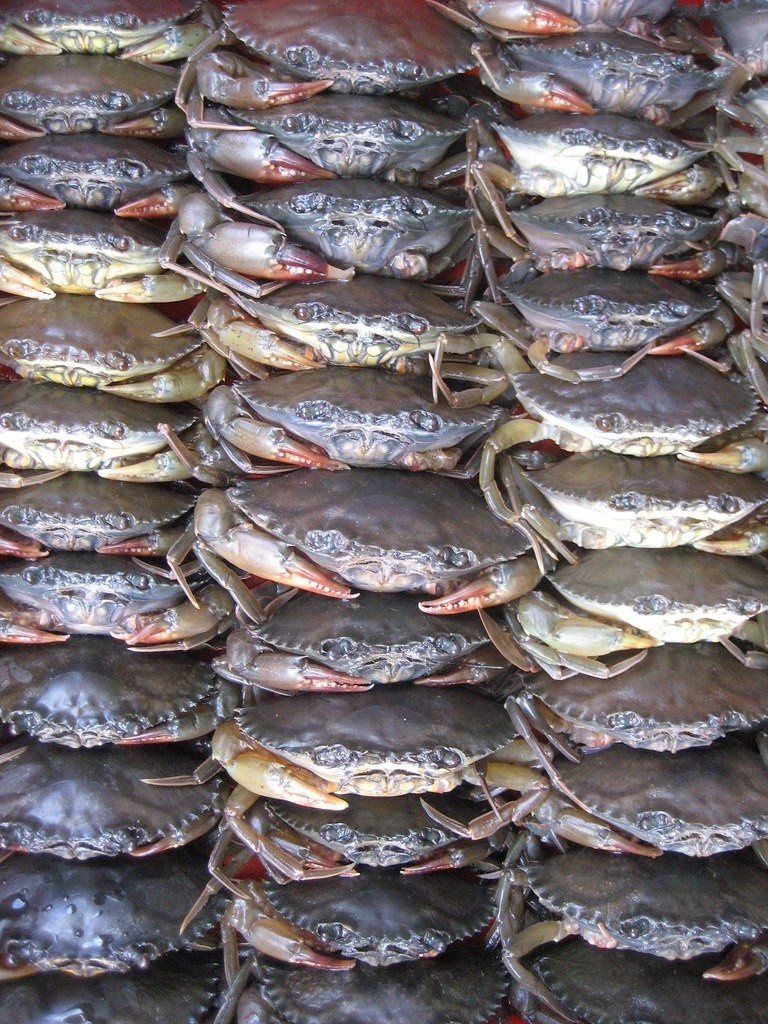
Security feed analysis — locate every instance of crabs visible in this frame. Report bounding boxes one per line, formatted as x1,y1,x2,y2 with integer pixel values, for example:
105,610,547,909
0,0,767,1024
